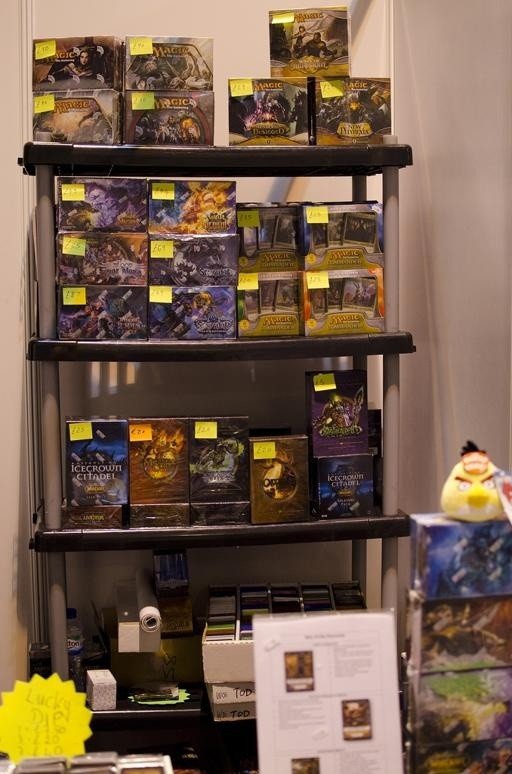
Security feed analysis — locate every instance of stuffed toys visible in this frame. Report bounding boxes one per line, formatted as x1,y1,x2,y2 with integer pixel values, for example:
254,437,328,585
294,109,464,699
442,440,511,521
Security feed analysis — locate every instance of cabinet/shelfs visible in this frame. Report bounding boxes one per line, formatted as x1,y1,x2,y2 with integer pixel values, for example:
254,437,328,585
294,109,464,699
20,142,414,720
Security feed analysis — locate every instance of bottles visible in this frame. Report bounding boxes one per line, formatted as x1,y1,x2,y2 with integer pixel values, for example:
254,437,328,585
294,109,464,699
66,607,86,692
